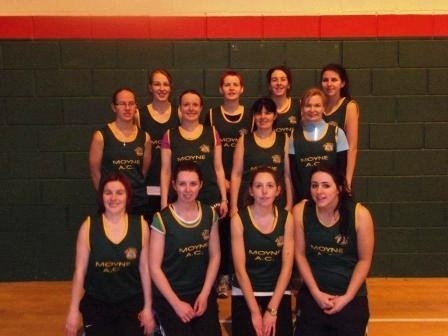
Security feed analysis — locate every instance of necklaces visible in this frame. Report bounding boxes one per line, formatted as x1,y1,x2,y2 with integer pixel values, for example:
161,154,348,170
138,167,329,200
113,124,135,148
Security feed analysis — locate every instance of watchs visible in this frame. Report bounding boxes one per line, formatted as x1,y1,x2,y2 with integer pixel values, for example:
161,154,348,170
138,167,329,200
266,308,277,316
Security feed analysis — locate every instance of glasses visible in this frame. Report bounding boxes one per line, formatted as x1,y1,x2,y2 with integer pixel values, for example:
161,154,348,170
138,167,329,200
114,102,138,107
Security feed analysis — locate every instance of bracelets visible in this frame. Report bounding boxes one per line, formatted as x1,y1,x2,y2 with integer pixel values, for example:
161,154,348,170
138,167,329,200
220,200,228,203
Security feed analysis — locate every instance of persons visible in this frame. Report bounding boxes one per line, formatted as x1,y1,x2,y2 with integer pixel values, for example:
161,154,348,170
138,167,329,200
89,86,152,215
148,163,221,336
288,89,351,204
203,70,257,176
161,88,228,219
136,67,183,170
230,96,292,218
65,173,155,336
230,166,294,336
252,65,339,159
320,64,360,196
293,161,373,336
